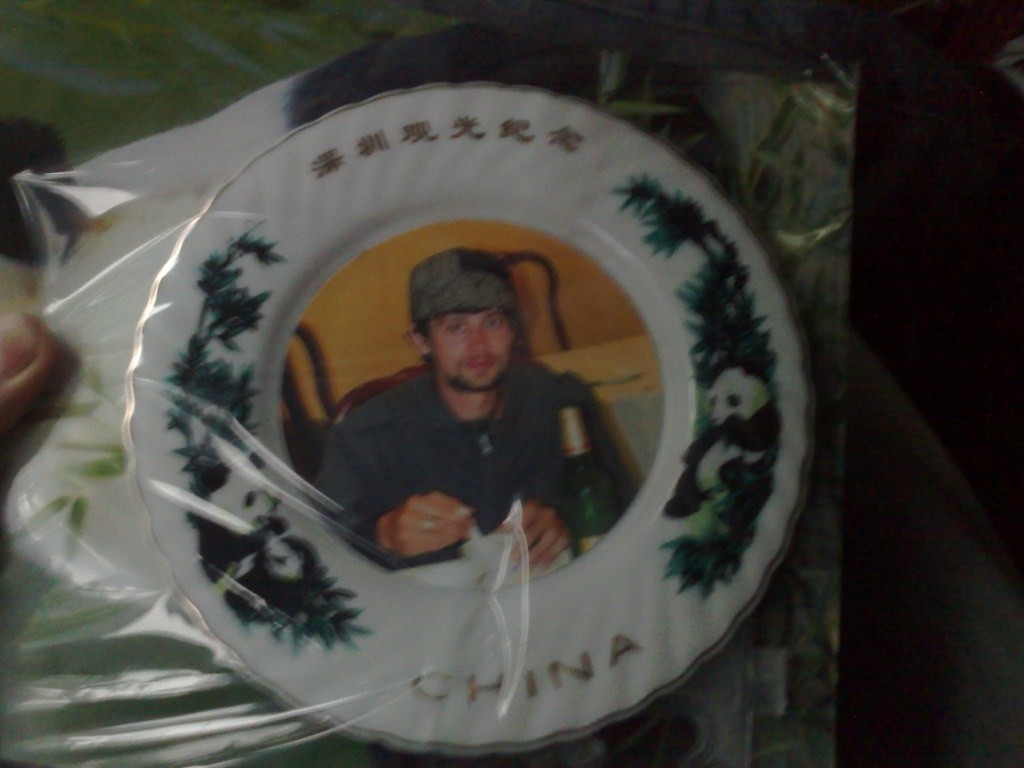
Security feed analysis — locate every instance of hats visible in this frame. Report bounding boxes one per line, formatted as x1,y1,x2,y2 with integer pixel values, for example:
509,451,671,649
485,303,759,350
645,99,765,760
408,247,518,323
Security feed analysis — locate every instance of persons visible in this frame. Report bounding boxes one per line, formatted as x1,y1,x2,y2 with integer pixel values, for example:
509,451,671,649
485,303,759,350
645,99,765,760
316,246,631,572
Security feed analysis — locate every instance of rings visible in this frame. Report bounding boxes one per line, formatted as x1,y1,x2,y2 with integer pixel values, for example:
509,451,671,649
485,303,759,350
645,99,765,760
424,522,434,530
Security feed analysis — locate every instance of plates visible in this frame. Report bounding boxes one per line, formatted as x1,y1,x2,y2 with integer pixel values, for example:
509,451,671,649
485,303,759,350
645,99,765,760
127,83,814,756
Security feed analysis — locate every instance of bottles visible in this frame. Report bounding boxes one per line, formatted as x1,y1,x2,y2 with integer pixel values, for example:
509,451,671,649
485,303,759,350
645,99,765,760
555,403,623,558
